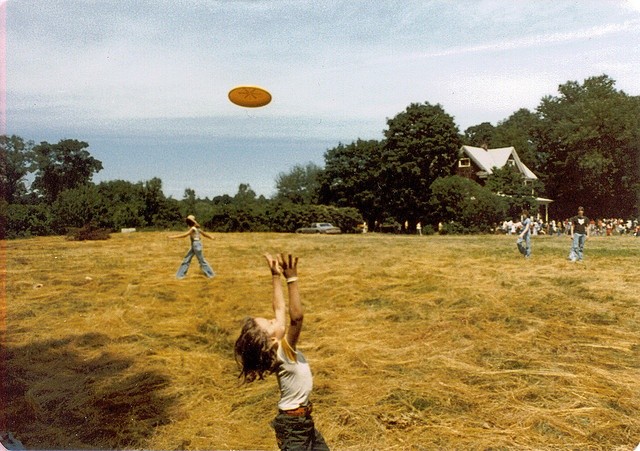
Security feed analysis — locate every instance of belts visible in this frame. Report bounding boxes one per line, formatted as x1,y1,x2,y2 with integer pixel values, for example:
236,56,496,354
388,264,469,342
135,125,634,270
280,400,313,418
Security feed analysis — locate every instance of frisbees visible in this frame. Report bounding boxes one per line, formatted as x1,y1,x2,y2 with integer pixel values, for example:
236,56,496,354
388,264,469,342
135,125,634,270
227,86,272,108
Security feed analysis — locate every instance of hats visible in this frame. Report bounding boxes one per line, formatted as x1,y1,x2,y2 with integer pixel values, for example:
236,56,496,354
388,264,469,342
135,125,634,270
183,214,200,228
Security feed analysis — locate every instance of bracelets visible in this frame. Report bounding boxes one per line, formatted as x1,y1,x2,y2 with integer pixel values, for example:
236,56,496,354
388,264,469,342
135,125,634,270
287,276,298,283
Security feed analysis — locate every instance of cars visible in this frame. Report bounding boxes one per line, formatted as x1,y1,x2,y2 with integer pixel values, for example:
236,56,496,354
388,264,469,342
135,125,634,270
295,221,342,233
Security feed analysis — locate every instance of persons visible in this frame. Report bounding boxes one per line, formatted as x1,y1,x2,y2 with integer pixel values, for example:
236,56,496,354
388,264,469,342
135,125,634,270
168,215,217,280
416,222,422,237
517,211,531,257
569,206,590,261
590,218,640,236
531,218,572,235
234,251,330,451
